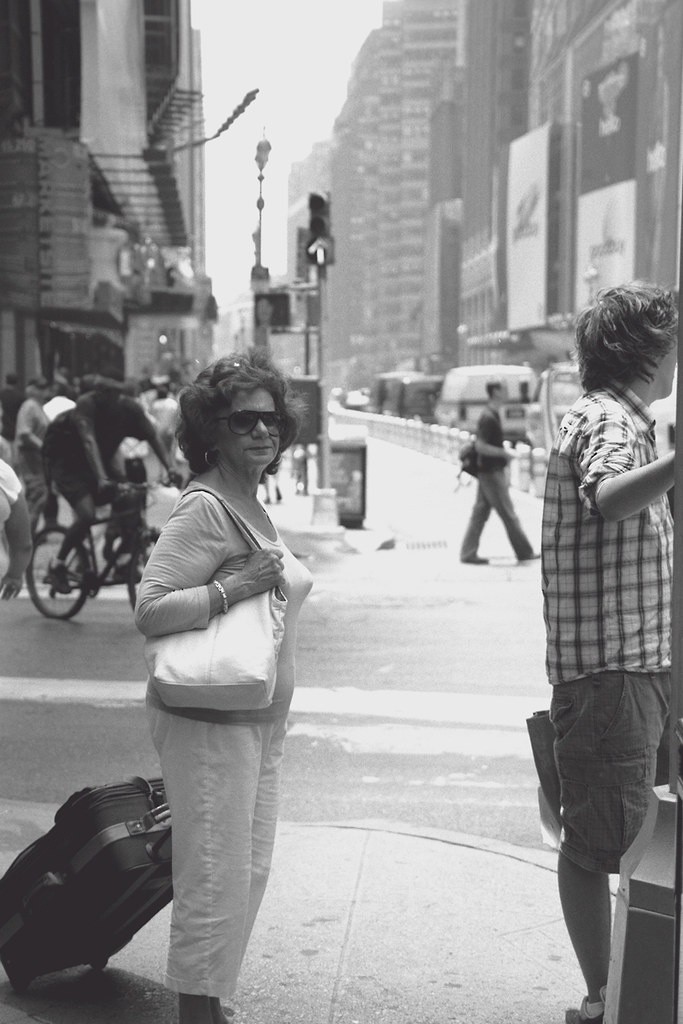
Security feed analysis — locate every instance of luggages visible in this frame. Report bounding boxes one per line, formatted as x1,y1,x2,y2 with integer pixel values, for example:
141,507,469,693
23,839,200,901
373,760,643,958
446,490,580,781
0,776,172,991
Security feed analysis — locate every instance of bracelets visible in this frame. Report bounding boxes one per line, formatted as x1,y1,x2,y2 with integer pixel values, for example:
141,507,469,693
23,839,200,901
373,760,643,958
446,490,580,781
213,580,228,615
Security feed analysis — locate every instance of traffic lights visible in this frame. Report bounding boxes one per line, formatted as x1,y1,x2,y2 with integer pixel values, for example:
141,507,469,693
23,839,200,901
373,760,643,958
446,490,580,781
305,187,334,265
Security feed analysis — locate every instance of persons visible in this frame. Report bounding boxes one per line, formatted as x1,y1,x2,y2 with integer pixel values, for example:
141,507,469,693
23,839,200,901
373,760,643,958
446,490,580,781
542,283,682,1024
459,375,543,564
1,356,282,598
135,357,312,1024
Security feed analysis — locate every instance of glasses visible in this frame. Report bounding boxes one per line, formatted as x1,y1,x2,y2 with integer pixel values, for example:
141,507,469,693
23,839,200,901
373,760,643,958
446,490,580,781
214,408,288,437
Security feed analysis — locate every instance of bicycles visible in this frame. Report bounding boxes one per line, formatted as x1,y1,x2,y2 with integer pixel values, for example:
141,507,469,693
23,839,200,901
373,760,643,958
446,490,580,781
26,474,162,621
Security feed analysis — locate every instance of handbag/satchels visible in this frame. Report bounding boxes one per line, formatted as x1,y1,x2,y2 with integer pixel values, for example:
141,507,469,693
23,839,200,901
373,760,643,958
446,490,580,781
456,440,478,478
142,586,288,710
524,707,682,824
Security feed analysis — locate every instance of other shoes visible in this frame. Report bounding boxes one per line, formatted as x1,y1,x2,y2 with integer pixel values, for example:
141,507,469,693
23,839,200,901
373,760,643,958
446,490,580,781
565,995,604,1024
464,551,489,563
45,559,68,579
518,552,542,560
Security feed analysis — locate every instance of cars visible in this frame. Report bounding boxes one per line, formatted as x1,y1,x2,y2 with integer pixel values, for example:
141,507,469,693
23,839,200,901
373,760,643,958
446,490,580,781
357,359,588,455
